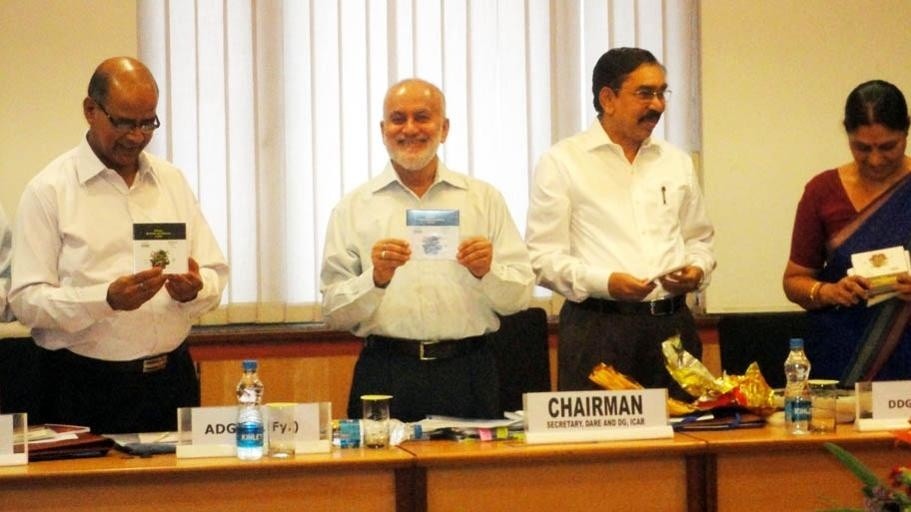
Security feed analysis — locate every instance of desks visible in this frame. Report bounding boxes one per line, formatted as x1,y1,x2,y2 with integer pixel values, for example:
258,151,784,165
1,443,414,512
394,432,707,512
684,422,911,512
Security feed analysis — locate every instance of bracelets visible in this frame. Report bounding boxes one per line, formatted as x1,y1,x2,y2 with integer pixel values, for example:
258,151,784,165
810,281,820,303
816,282,826,300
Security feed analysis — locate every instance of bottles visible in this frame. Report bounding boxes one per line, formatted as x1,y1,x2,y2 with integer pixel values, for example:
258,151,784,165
332,419,422,449
237,361,264,460
784,338,811,434
339,419,360,449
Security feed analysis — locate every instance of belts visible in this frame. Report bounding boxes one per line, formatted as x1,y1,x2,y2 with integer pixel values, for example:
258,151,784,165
90,341,192,373
570,299,686,317
363,337,484,361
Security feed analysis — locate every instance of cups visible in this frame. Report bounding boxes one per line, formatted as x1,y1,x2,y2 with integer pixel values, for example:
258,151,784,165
361,394,392,448
808,379,839,433
268,406,294,457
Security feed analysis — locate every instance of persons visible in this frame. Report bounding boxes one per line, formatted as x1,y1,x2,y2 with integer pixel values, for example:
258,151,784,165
321,81,534,419
0,210,18,322
783,79,911,389
7,55,231,433
524,47,717,404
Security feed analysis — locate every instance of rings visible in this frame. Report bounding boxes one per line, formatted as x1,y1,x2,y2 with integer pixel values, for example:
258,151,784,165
380,251,385,258
139,282,145,292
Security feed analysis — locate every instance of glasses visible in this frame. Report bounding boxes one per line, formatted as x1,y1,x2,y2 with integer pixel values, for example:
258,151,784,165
608,86,674,99
92,99,161,133
